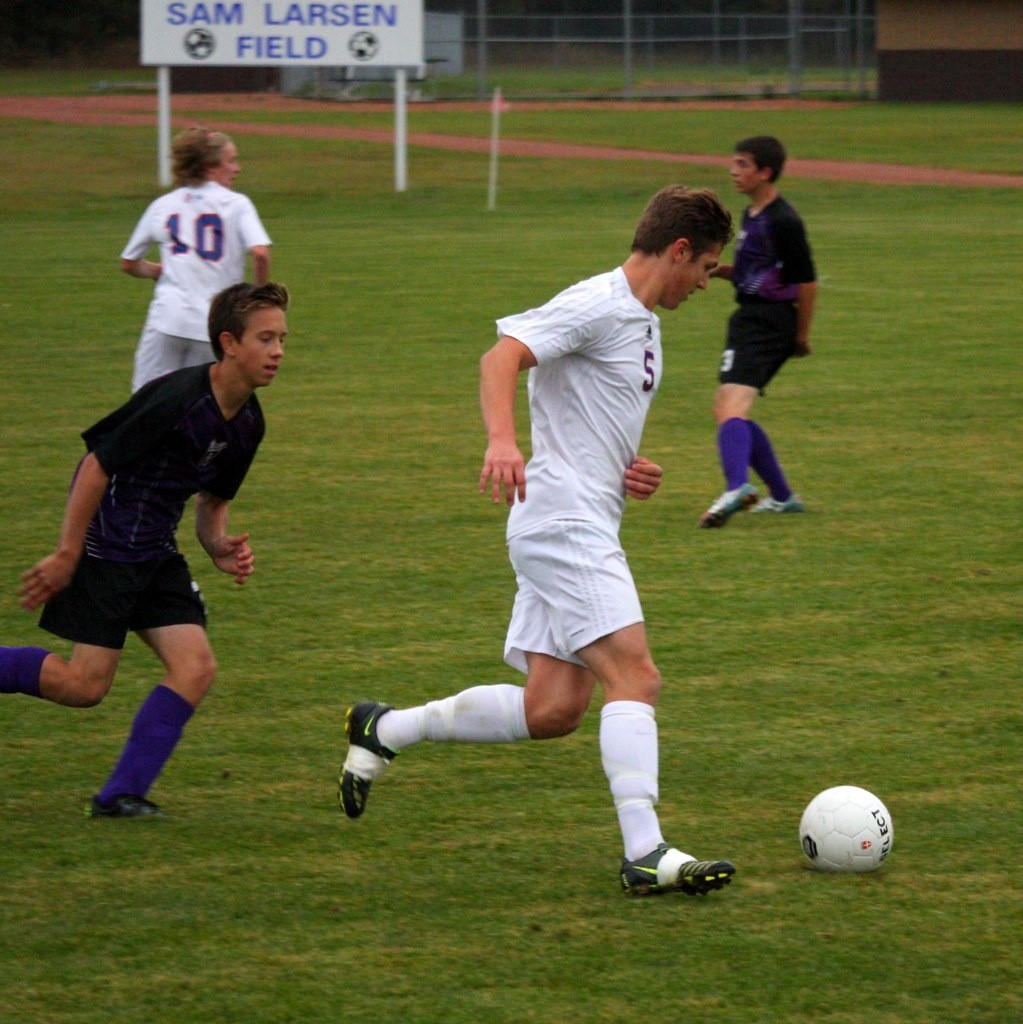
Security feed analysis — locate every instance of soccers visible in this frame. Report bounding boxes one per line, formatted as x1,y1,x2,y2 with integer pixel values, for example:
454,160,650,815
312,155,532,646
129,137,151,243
799,786,894,873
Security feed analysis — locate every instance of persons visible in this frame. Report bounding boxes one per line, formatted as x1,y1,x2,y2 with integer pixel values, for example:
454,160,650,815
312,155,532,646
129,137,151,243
119,123,271,402
338,185,738,899
0,282,289,814
697,135,816,528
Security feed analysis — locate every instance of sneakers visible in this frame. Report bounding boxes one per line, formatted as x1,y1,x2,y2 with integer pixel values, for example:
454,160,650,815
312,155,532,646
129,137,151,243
750,492,805,512
617,841,737,897
697,482,759,527
337,699,400,820
89,793,162,818
